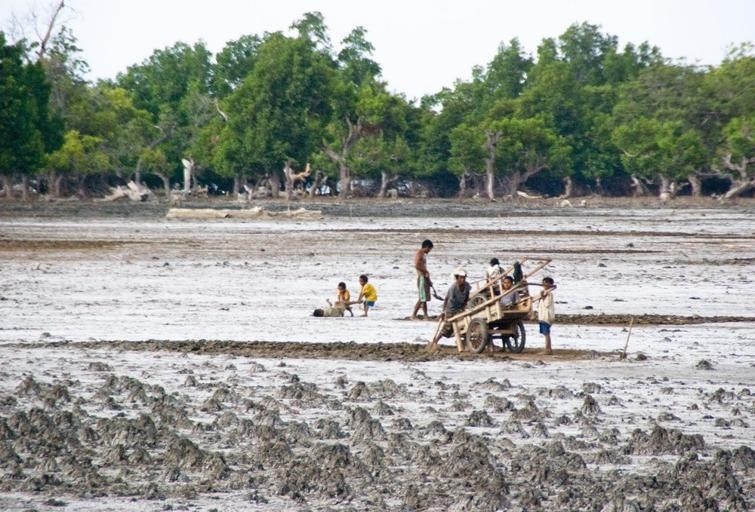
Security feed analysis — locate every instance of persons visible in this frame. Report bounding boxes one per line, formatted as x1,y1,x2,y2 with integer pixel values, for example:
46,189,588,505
357,274,377,317
486,258,518,351
498,276,520,308
427,270,471,353
336,282,355,317
538,277,556,355
313,298,345,317
410,240,435,321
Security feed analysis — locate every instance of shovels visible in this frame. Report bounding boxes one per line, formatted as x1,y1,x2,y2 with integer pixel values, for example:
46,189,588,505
425,318,443,353
427,277,444,301
620,316,635,358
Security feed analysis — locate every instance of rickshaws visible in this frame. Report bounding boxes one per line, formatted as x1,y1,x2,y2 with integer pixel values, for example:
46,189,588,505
435,255,556,354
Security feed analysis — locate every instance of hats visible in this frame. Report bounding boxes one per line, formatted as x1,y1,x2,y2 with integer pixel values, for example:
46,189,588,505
454,268,468,277
